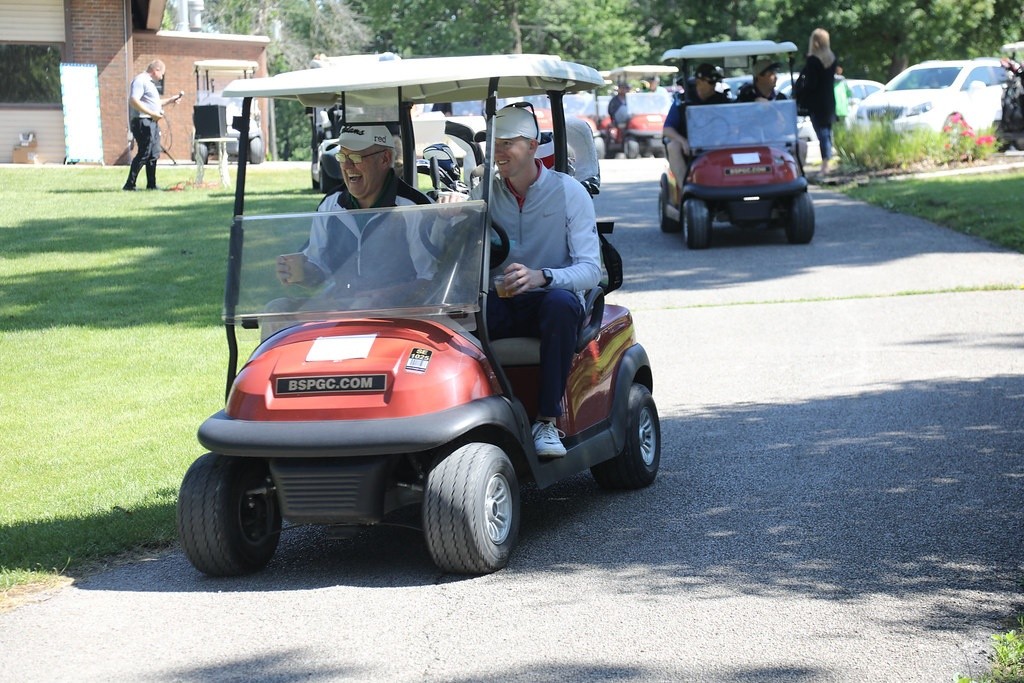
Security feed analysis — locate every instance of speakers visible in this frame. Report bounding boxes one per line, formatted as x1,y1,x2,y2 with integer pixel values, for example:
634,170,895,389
194,105,227,138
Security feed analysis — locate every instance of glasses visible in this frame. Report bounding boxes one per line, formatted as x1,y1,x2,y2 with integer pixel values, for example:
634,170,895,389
699,76,722,85
503,99,540,142
334,149,387,164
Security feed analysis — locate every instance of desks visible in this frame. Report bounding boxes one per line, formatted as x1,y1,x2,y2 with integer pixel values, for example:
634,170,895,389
195,137,238,189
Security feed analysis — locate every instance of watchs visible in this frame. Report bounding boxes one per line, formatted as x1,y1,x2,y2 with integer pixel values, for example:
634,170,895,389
540,269,553,287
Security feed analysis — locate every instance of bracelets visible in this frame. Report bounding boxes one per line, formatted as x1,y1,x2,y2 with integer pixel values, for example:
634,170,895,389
437,213,453,221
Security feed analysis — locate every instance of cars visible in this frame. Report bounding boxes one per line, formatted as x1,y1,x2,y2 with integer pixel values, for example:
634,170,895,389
845,57,1016,166
718,71,885,163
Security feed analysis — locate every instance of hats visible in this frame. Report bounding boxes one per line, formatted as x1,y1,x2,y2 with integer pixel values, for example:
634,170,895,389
618,81,631,88
752,58,784,80
495,100,542,144
336,126,398,151
696,63,725,81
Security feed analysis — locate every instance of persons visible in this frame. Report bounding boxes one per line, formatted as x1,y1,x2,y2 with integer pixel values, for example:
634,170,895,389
258,124,438,348
429,101,602,455
663,64,731,191
608,77,668,127
793,28,837,177
737,61,807,172
1000,58,1024,86
123,58,182,191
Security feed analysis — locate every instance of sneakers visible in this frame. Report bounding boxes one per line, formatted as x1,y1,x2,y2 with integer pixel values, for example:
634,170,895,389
532,421,567,456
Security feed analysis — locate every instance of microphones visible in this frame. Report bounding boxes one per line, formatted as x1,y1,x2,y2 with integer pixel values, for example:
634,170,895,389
174,92,184,106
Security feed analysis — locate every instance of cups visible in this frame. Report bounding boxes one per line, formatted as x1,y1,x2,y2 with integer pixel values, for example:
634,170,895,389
493,273,519,299
280,252,305,283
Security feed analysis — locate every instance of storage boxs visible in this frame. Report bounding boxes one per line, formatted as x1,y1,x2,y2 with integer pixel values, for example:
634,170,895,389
12,145,38,164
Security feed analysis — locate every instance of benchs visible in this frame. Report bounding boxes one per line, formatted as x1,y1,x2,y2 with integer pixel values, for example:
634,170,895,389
488,220,623,366
